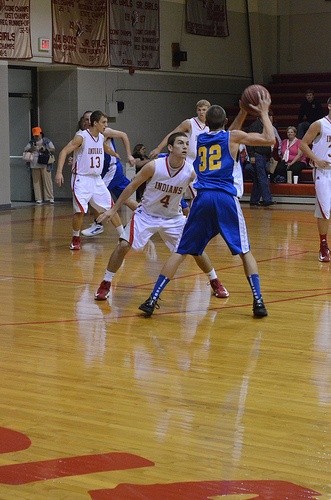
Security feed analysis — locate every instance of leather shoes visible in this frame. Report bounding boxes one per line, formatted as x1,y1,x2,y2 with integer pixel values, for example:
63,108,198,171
264,201,276,206
250,201,262,206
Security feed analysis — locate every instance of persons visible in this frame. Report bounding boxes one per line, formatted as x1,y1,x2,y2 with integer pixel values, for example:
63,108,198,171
296,89,324,139
56,110,125,250
245,109,277,206
239,144,253,181
94,132,229,300
280,126,307,184
299,98,331,262
133,143,150,174
25,127,56,204
138,90,276,317
68,111,139,236
150,99,210,197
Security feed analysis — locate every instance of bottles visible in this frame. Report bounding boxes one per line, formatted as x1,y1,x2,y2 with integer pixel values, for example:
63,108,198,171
286,169,292,184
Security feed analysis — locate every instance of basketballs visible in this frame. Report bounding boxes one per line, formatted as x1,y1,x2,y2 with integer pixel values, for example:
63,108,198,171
241,84,270,113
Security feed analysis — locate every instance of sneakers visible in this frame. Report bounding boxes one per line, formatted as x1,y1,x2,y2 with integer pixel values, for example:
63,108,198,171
206,278,230,297
94,280,112,300
69,236,81,249
254,305,267,317
318,247,331,262
139,298,160,313
82,219,105,236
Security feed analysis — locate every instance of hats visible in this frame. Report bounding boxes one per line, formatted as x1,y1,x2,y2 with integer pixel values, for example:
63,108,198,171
32,127,42,136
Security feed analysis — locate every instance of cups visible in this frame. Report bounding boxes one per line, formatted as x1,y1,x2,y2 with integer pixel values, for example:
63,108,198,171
293,176,298,184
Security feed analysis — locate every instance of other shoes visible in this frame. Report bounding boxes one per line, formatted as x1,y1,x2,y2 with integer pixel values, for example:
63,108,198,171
37,200,42,204
49,200,55,204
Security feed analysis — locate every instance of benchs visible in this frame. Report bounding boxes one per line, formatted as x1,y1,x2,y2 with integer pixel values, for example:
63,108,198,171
232,69,330,197
